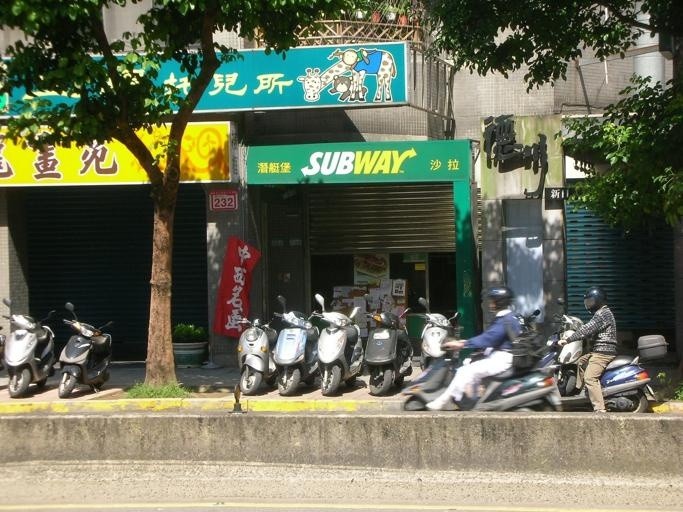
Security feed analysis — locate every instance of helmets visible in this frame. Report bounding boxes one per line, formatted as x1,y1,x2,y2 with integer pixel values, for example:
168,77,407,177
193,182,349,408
583,288,607,314
487,286,513,301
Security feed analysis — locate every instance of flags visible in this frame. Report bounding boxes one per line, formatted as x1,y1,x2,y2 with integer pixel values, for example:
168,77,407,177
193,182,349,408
213,236,261,341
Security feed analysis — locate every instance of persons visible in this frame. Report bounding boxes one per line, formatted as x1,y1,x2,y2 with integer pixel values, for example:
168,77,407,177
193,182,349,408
553,285,619,412
423,287,528,412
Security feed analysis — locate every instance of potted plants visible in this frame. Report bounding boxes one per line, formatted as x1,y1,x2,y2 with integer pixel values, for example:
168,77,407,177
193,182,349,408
354,0,412,25
171,322,209,367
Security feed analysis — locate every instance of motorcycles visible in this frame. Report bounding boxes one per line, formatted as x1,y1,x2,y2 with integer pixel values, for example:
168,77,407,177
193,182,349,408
0,297,115,398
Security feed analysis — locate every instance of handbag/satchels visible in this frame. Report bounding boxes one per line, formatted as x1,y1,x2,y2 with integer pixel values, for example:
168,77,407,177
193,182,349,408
512,333,544,377
